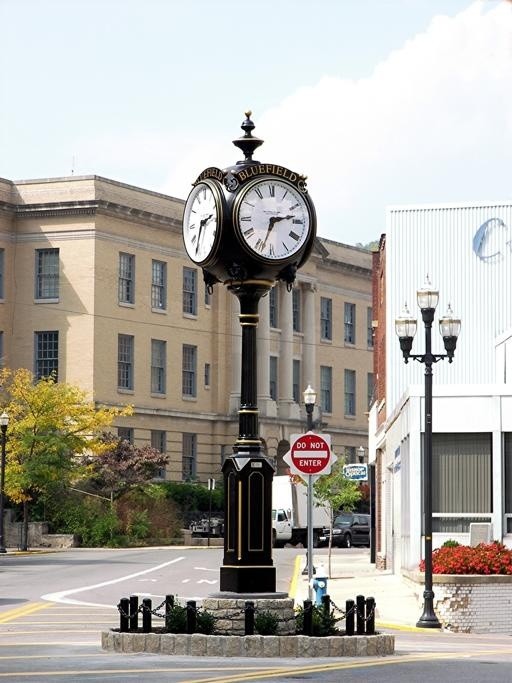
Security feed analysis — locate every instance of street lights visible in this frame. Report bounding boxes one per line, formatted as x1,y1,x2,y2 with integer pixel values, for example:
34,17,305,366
385,268,465,635
300,383,320,577
355,445,368,513
0,409,13,553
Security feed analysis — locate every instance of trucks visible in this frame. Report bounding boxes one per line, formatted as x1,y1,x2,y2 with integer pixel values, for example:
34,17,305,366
266,474,334,550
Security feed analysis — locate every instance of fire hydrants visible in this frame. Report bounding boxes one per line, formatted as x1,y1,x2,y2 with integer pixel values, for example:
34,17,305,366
310,574,330,611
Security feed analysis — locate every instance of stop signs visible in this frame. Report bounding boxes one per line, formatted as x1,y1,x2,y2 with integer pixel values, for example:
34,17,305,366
288,433,333,476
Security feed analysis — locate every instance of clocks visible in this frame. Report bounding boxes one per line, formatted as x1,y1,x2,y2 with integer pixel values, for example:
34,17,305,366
179,108,319,596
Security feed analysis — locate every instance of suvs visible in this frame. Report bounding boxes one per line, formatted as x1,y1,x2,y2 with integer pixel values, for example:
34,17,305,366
318,510,370,549
